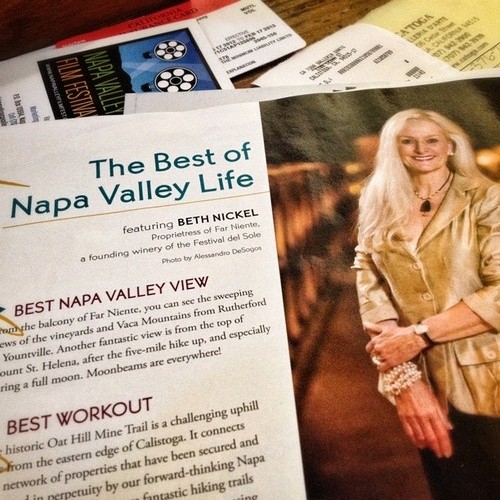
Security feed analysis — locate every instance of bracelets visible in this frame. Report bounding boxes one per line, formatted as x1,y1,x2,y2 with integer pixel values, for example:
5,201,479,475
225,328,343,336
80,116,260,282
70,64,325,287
382,362,423,397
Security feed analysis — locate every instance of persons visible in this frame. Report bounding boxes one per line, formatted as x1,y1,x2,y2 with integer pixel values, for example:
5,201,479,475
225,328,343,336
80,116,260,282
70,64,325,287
352,109,499,500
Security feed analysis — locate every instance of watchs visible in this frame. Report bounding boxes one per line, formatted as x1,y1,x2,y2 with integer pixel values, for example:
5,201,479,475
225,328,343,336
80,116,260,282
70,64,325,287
414,320,436,347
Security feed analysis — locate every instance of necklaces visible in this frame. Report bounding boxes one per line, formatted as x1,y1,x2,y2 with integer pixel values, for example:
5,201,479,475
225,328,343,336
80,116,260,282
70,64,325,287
411,169,453,217
